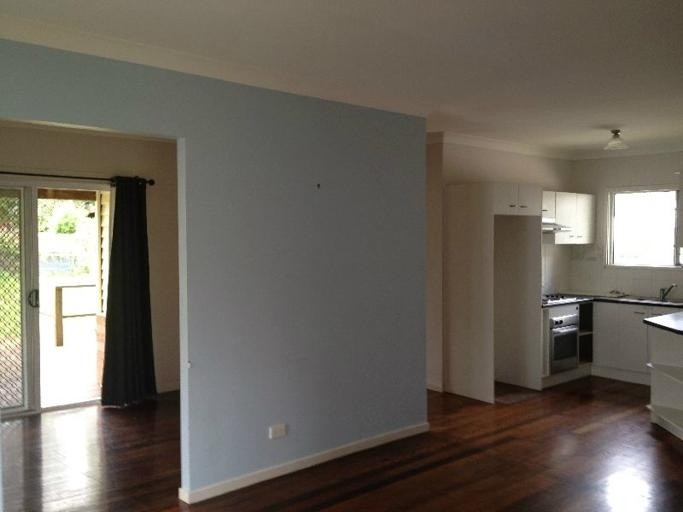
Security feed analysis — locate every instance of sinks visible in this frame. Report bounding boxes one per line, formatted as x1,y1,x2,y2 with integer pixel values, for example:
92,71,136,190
638,299,671,301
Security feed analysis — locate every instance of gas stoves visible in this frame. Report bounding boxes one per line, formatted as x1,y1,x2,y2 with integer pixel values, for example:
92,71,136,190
543,294,592,304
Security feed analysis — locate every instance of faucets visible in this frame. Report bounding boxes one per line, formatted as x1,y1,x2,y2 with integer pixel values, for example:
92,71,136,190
660,284,678,301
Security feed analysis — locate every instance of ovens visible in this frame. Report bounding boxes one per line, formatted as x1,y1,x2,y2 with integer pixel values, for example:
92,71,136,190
542,304,579,374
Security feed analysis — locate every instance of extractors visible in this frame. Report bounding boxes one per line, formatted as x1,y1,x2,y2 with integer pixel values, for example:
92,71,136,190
541,217,572,235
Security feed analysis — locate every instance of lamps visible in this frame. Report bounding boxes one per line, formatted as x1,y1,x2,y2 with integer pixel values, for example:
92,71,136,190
601,128,629,151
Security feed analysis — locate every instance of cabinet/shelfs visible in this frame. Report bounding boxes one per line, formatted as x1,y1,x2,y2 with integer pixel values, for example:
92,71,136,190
444,179,545,406
542,191,596,245
642,309,682,440
591,300,621,382
622,302,679,385
